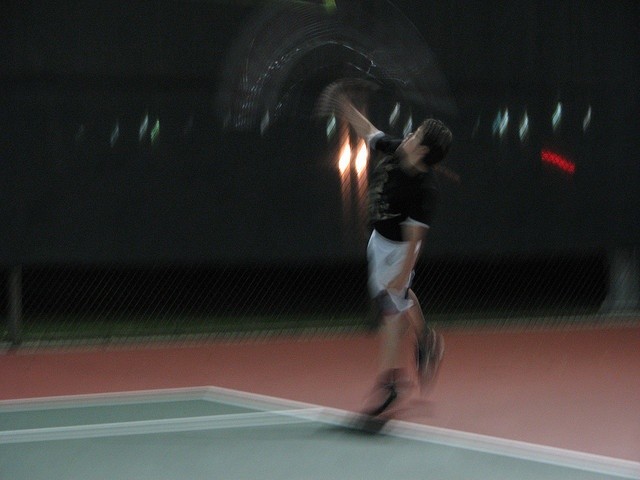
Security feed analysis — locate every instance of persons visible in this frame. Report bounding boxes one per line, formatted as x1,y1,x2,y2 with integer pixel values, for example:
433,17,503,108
318,81,453,420
